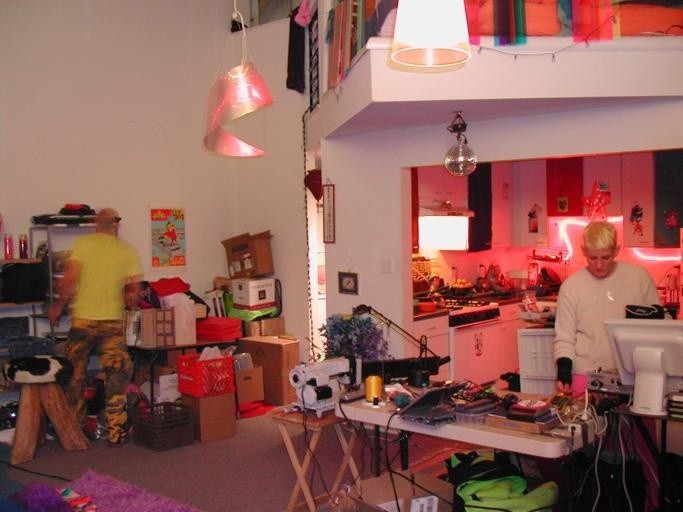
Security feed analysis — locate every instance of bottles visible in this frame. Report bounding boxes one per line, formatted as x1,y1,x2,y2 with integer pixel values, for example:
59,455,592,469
3,234,13,259
18,234,27,259
365,373,382,403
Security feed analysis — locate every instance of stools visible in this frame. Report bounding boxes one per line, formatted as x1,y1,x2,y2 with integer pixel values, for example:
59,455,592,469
0,350,91,466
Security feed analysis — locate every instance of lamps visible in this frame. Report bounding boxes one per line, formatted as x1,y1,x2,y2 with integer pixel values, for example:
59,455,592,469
201,0,276,159
390,0,473,69
441,107,477,176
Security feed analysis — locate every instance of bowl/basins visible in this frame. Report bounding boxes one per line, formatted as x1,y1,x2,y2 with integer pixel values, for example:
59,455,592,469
449,287,473,296
418,302,435,312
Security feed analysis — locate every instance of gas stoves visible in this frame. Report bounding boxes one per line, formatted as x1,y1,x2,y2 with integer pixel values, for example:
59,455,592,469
435,299,500,326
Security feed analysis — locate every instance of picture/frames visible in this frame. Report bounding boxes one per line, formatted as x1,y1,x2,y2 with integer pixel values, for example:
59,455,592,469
337,270,358,295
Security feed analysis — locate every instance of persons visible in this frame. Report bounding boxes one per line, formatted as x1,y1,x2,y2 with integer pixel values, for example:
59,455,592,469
47,206,145,444
553,220,666,395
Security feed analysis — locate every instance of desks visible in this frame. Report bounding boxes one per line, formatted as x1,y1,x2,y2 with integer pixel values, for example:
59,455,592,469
334,381,629,511
525,317,555,328
606,401,682,512
269,407,362,512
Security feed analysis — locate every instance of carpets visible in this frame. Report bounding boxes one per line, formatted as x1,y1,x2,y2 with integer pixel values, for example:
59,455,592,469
52,470,203,512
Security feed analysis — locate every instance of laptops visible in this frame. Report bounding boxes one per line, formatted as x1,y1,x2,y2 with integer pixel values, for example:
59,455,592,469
400,386,463,426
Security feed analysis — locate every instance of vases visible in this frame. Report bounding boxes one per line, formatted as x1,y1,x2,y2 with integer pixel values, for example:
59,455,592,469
346,353,357,385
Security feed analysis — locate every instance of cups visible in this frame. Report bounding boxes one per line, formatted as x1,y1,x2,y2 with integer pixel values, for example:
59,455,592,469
524,291,536,311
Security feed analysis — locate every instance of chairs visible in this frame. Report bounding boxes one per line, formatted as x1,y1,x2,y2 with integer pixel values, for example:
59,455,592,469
516,328,563,396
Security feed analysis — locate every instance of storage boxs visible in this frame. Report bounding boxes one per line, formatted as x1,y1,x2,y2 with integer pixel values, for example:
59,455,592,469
343,468,455,511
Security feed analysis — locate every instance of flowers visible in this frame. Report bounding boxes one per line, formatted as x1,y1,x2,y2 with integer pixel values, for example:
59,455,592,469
316,311,394,367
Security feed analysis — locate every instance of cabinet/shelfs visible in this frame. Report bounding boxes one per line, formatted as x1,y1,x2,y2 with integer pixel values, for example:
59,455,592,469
0,253,48,309
412,158,560,252
499,300,555,373
546,155,623,217
623,148,683,249
27,222,123,352
414,311,449,382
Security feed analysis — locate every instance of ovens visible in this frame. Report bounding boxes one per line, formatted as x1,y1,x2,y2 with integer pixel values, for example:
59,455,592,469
447,318,503,386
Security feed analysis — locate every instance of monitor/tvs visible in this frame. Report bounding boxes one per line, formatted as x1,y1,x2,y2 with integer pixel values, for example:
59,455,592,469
605,319,683,416
0,263,48,303
